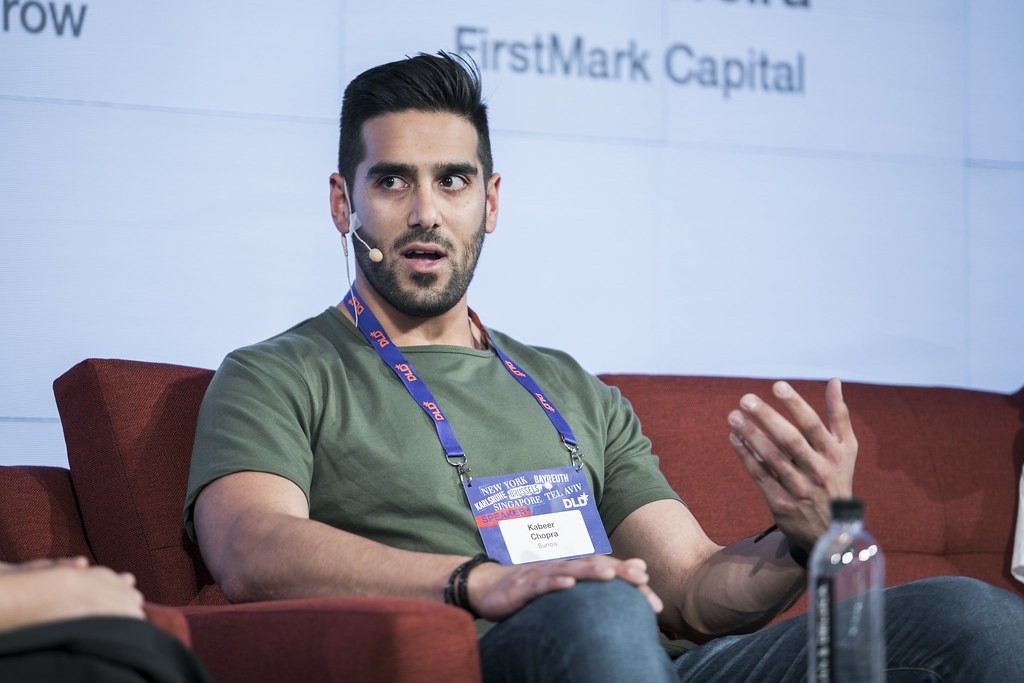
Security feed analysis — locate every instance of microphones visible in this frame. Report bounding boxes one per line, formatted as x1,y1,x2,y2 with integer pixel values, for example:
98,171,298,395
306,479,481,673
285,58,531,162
351,230,383,262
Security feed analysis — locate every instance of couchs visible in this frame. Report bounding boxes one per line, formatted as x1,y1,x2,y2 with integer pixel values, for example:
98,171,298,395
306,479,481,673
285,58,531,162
0,358,1024,683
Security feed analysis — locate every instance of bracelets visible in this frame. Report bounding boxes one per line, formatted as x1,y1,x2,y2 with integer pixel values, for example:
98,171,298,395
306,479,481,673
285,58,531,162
444,554,501,619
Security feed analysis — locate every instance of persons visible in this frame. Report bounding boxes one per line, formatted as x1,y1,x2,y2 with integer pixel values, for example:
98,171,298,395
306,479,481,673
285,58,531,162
0,556,213,683
181,55,1024,683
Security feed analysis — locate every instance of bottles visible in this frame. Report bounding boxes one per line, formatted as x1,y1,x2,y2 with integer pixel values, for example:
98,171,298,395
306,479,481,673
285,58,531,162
808,499,888,683
